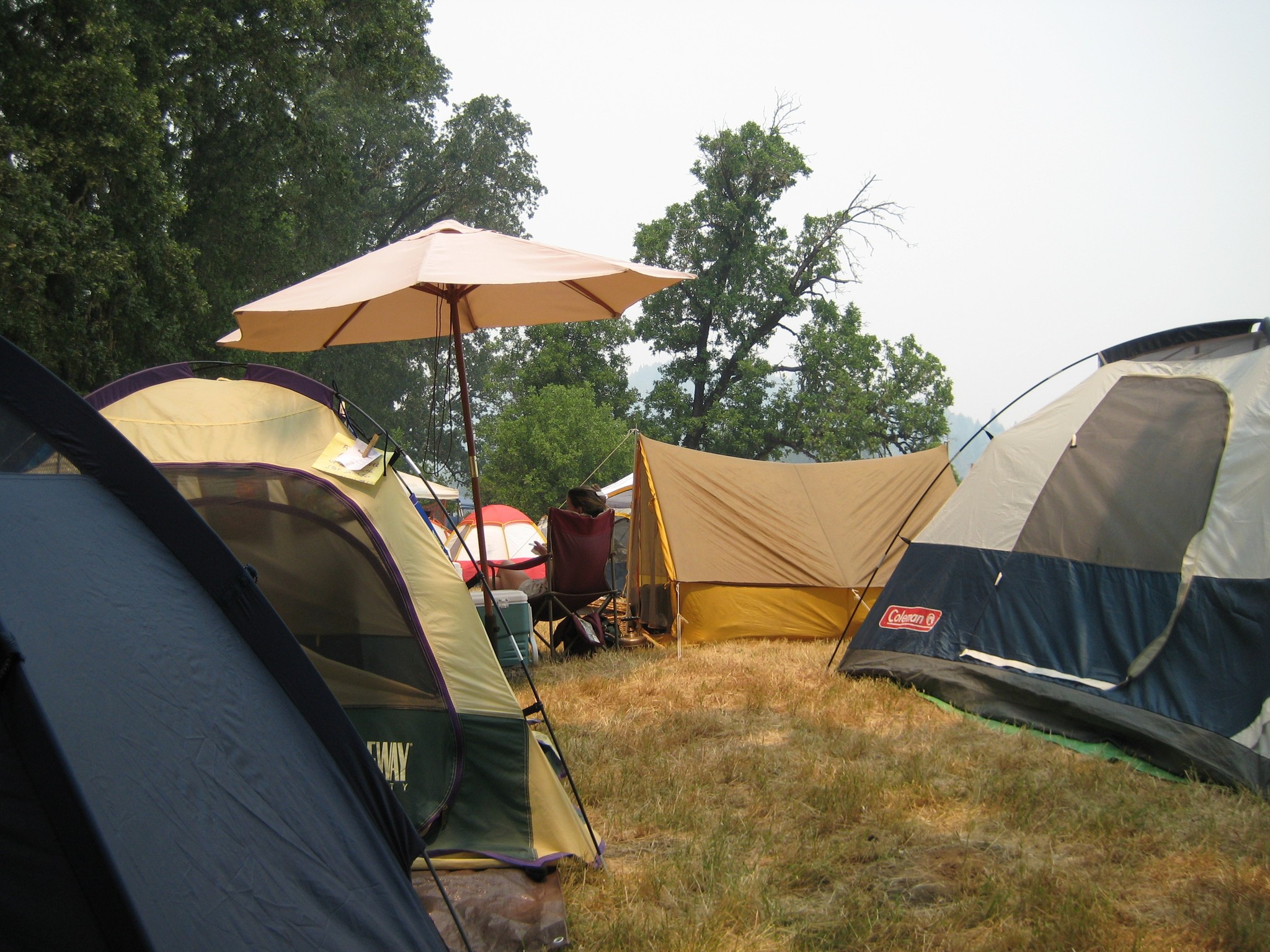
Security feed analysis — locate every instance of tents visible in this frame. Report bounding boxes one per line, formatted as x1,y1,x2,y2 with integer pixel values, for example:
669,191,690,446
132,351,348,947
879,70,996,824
826,318,1270,805
0,360,956,952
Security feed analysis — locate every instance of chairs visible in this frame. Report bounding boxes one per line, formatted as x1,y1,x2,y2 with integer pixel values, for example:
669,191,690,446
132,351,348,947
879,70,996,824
477,506,623,666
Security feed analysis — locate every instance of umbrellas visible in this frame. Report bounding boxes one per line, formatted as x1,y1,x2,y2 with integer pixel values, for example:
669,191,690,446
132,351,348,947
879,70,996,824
216,217,698,657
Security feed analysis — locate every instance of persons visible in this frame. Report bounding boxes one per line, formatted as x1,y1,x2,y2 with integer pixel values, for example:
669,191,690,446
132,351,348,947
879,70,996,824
477,485,606,619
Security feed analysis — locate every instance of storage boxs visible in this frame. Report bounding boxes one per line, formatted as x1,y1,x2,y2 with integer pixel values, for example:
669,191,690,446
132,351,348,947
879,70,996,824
471,589,539,668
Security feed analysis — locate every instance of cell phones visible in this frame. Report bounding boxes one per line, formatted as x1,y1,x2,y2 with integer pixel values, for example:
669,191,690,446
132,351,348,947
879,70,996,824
528,543,536,546
